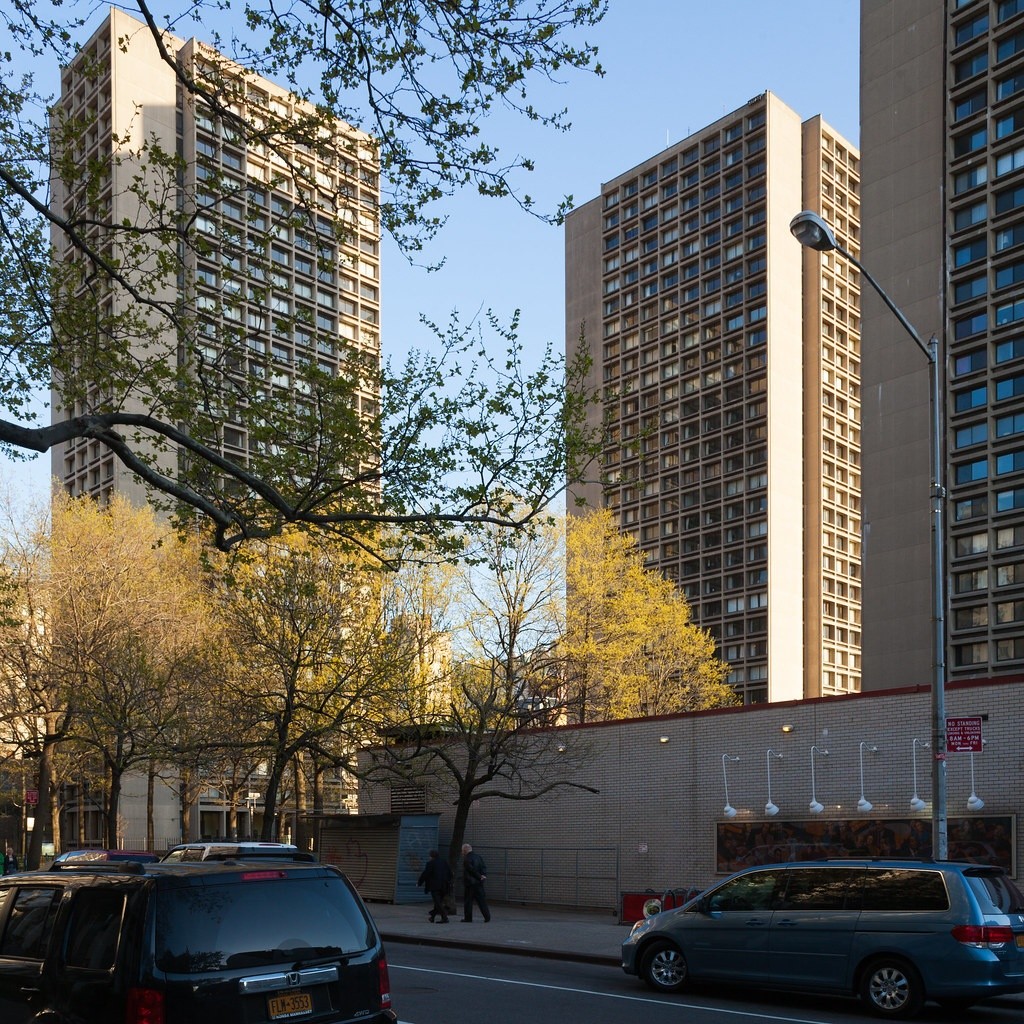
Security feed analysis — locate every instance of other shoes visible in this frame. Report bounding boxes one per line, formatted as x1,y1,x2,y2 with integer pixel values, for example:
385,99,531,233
428,917,434,923
461,918,472,922
484,917,490,922
436,918,449,923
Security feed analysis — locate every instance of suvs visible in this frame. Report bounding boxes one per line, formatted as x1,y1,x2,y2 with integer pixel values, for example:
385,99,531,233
623,860,1024,1018
56,850,159,863
158,842,298,862
0,852,398,1024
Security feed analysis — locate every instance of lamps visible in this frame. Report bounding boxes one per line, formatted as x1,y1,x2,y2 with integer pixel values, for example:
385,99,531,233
721,753,742,816
557,746,568,752
909,738,932,812
855,740,879,812
765,749,784,817
810,745,830,813
965,738,989,811
782,724,794,732
660,735,670,743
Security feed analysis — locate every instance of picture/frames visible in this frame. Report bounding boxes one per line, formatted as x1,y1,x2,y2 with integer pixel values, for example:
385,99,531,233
711,815,1017,882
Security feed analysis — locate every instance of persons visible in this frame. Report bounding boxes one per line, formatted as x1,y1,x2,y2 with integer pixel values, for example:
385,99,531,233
3,848,19,876
416,849,454,924
460,845,494,925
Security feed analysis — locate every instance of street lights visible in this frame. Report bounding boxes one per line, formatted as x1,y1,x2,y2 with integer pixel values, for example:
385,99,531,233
790,212,945,860
